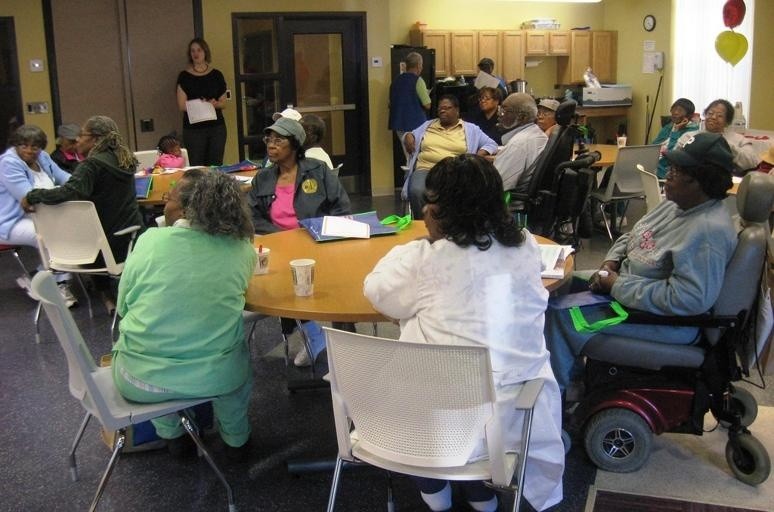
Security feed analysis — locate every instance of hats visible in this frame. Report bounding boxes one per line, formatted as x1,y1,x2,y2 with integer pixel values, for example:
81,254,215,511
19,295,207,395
537,98,561,112
261,117,307,148
662,133,733,174
272,108,303,122
58,124,80,139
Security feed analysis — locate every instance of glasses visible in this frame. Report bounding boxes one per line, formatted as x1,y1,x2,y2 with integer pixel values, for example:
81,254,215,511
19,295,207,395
497,104,529,118
76,131,100,139
437,105,455,113
665,160,688,177
477,96,495,102
262,136,289,146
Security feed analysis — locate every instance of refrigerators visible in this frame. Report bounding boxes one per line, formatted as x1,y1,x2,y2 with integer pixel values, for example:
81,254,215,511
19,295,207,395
389,45,436,188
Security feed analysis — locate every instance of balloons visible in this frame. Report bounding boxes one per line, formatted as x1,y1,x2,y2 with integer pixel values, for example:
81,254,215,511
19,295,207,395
714,0,752,65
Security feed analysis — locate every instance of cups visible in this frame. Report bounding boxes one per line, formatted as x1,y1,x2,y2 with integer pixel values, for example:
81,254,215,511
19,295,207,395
252,248,270,274
288,258,315,296
616,137,627,149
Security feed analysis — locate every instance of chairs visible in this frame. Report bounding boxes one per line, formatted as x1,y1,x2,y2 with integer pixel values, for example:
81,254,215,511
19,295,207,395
0,97,772,512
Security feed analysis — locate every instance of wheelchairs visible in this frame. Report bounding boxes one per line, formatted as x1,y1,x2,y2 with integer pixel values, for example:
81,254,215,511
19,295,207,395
559,170,774,486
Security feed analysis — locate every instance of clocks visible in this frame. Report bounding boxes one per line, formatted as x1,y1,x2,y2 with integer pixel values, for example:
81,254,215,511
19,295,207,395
642,14,656,32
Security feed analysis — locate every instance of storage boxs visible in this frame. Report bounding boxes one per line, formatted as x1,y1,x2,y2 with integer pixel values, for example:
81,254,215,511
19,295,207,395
576,85,632,107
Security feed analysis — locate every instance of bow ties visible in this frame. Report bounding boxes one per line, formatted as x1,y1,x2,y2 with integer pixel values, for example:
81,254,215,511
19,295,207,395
322,372,330,382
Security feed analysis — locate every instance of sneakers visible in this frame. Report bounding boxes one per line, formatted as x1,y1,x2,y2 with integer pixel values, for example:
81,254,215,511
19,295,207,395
420,481,452,511
56,281,79,309
16,272,41,303
293,326,325,368
594,209,628,228
466,494,499,511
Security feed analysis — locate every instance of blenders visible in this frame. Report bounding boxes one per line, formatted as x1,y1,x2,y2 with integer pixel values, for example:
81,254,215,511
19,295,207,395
510,79,527,93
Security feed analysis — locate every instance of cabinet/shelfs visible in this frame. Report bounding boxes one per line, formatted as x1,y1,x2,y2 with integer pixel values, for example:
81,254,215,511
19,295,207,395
557,30,617,85
409,30,477,77
477,30,524,84
524,30,570,56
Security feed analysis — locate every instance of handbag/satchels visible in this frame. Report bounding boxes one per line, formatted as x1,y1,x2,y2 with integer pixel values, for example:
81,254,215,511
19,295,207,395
101,353,169,454
547,289,616,311
